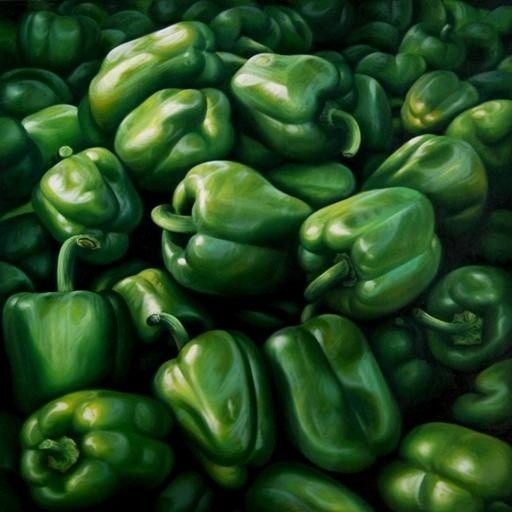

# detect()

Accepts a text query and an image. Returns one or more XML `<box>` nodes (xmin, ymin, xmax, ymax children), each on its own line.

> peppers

<box><xmin>0</xmin><ymin>0</ymin><xmax>510</xmax><ymax>512</ymax></box>
